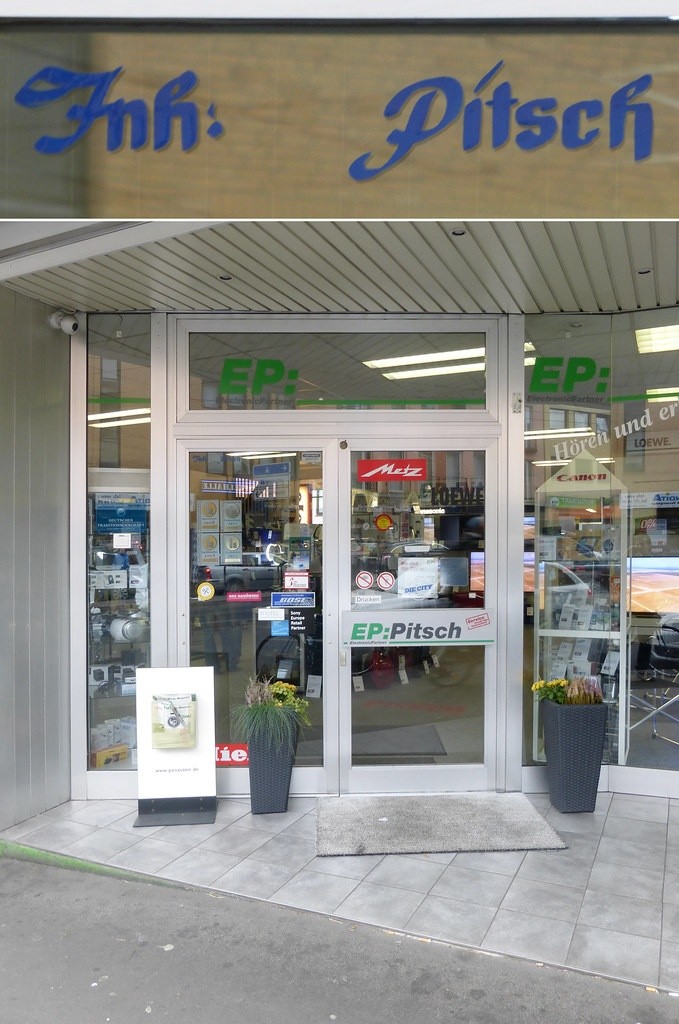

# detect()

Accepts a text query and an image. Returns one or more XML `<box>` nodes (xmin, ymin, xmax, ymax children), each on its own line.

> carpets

<box><xmin>352</xmin><ymin>723</ymin><xmax>447</xmax><ymax>756</ymax></box>
<box><xmin>315</xmin><ymin>793</ymin><xmax>567</xmax><ymax>856</ymax></box>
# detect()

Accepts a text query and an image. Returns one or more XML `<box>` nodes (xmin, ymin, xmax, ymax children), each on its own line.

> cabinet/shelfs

<box><xmin>533</xmin><ymin>449</ymin><xmax>633</xmax><ymax>765</ymax></box>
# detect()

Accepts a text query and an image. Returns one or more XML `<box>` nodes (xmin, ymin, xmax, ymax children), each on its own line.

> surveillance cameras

<box><xmin>61</xmin><ymin>315</ymin><xmax>80</xmax><ymax>335</ymax></box>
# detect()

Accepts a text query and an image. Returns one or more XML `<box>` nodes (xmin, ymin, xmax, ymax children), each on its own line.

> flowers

<box><xmin>531</xmin><ymin>678</ymin><xmax>602</xmax><ymax>706</ymax></box>
<box><xmin>226</xmin><ymin>673</ymin><xmax>312</xmax><ymax>755</ymax></box>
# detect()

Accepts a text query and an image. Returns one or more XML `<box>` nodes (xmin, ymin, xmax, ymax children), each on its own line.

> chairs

<box><xmin>625</xmin><ymin>622</ymin><xmax>679</xmax><ymax>746</ymax></box>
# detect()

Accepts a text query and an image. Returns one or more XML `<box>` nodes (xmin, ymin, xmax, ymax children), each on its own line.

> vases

<box><xmin>543</xmin><ymin>699</ymin><xmax>609</xmax><ymax>813</ymax></box>
<box><xmin>248</xmin><ymin>713</ymin><xmax>301</xmax><ymax>814</ymax></box>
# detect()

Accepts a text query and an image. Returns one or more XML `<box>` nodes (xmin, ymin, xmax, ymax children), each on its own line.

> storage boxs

<box><xmin>89</xmin><ymin>563</ymin><xmax>149</xmax><ymax>590</ymax></box>
<box><xmin>540</xmin><ymin>516</ymin><xmax>620</xmax><ymax>681</ymax></box>
<box><xmin>196</xmin><ymin>500</ymin><xmax>243</xmax><ymax>565</ymax></box>
<box><xmin>351</xmin><ymin>513</ymin><xmax>423</xmax><ymax>544</ymax></box>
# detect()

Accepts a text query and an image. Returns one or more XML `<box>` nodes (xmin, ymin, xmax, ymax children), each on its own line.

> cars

<box><xmin>543</xmin><ymin>564</ymin><xmax>594</xmax><ymax>625</ymax></box>
<box><xmin>93</xmin><ymin>550</ymin><xmax>136</xmax><ymax>600</ymax></box>
<box><xmin>193</xmin><ymin>552</ymin><xmax>293</xmax><ymax>594</ymax></box>
<box><xmin>367</xmin><ymin>539</ymin><xmax>450</xmax><ymax>576</ymax></box>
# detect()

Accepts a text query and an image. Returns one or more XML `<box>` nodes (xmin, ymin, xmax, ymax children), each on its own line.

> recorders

<box><xmin>109</xmin><ymin>617</ymin><xmax>151</xmax><ymax>643</ymax></box>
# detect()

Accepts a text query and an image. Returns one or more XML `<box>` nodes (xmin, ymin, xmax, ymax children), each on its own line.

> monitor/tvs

<box><xmin>524</xmin><ymin>516</ymin><xmax>535</xmax><ymax>549</ymax></box>
<box><xmin>467</xmin><ymin>551</ymin><xmax>535</xmax><ymax>594</ymax></box>
<box><xmin>626</xmin><ymin>556</ymin><xmax>679</xmax><ymax>619</ymax></box>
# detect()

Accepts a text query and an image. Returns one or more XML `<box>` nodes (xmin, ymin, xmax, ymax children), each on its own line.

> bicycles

<box><xmin>602</xmin><ymin>607</ymin><xmax>679</xmax><ymax>678</ymax></box>
<box><xmin>255</xmin><ymin>605</ymin><xmax>385</xmax><ymax>688</ymax></box>
